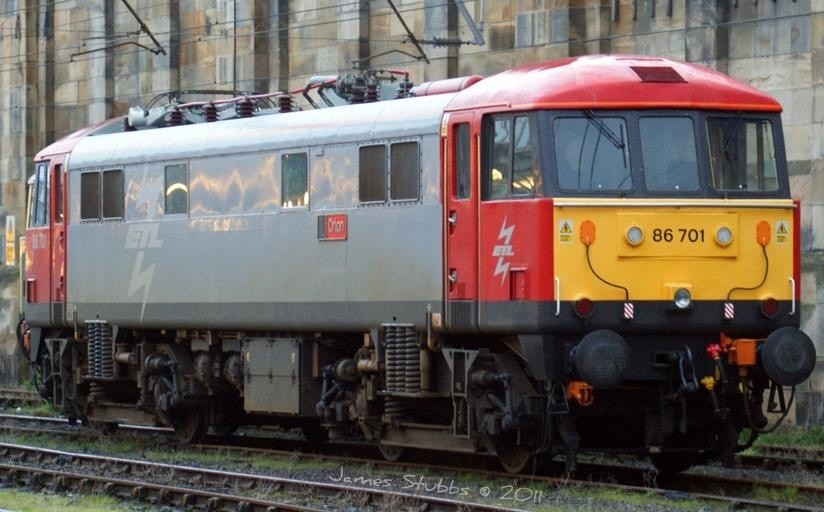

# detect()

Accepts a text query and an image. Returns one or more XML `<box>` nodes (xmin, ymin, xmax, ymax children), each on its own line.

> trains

<box><xmin>18</xmin><ymin>53</ymin><xmax>822</xmax><ymax>477</ymax></box>
